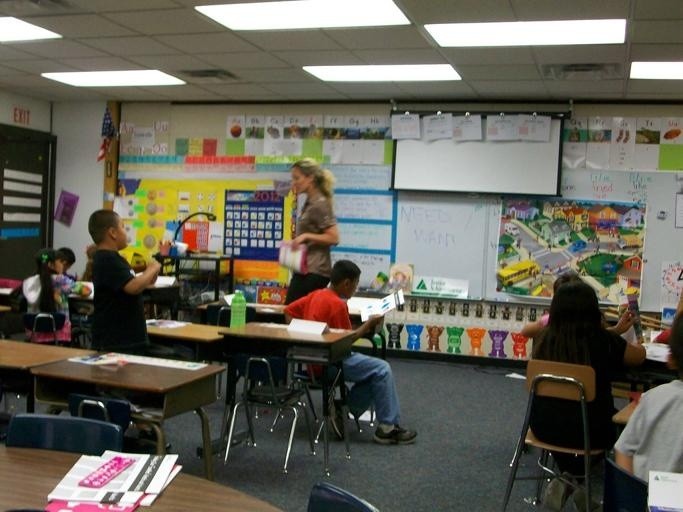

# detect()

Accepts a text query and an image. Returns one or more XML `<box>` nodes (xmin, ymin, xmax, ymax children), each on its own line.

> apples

<box><xmin>231</xmin><ymin>126</ymin><xmax>241</xmax><ymax>138</ymax></box>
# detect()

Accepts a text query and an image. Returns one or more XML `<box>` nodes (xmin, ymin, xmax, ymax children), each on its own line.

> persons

<box><xmin>284</xmin><ymin>156</ymin><xmax>340</xmax><ymax>305</ymax></box>
<box><xmin>88</xmin><ymin>210</ymin><xmax>171</xmax><ymax>450</ymax></box>
<box><xmin>521</xmin><ymin>272</ymin><xmax>646</xmax><ymax>510</ymax></box>
<box><xmin>22</xmin><ymin>247</ymin><xmax>92</xmax><ymax>348</ymax></box>
<box><xmin>613</xmin><ymin>311</ymin><xmax>683</xmax><ymax>489</ymax></box>
<box><xmin>284</xmin><ymin>260</ymin><xmax>418</xmax><ymax>446</ymax></box>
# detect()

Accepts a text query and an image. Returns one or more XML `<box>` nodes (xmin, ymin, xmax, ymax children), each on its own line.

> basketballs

<box><xmin>230</xmin><ymin>124</ymin><xmax>242</xmax><ymax>137</ymax></box>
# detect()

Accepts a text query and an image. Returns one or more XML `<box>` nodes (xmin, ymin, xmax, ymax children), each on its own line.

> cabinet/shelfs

<box><xmin>152</xmin><ymin>251</ymin><xmax>235</xmax><ymax>320</ymax></box>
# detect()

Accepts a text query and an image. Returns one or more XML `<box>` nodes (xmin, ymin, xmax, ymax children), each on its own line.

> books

<box><xmin>279</xmin><ymin>241</ymin><xmax>310</xmax><ymax>276</ymax></box>
<box><xmin>44</xmin><ymin>450</ymin><xmax>184</xmax><ymax>512</ymax></box>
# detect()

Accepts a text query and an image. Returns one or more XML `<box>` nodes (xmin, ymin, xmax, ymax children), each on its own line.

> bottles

<box><xmin>229</xmin><ymin>289</ymin><xmax>246</xmax><ymax>328</ymax></box>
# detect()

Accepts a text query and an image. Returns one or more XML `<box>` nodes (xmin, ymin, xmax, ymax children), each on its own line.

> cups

<box><xmin>170</xmin><ymin>246</ymin><xmax>178</xmax><ymax>256</ymax></box>
<box><xmin>159</xmin><ymin>245</ymin><xmax>170</xmax><ymax>256</ymax></box>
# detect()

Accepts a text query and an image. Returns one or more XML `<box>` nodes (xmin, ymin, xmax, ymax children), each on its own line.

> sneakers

<box><xmin>327</xmin><ymin>398</ymin><xmax>353</xmax><ymax>423</ymax></box>
<box><xmin>543</xmin><ymin>472</ymin><xmax>599</xmax><ymax>510</ymax></box>
<box><xmin>374</xmin><ymin>423</ymin><xmax>418</xmax><ymax>445</ymax></box>
<box><xmin>139</xmin><ymin>429</ymin><xmax>170</xmax><ymax>450</ymax></box>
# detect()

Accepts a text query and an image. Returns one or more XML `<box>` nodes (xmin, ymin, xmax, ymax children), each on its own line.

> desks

<box><xmin>612</xmin><ymin>398</ymin><xmax>637</xmax><ymax>423</ymax></box>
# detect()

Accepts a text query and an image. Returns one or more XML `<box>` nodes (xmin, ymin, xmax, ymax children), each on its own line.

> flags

<box><xmin>96</xmin><ymin>109</ymin><xmax>115</xmax><ymax>162</ymax></box>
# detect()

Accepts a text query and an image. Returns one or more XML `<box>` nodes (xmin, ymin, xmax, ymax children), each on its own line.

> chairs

<box><xmin>603</xmin><ymin>448</ymin><xmax>647</xmax><ymax>510</ymax></box>
<box><xmin>496</xmin><ymin>358</ymin><xmax>612</xmax><ymax>512</ymax></box>
